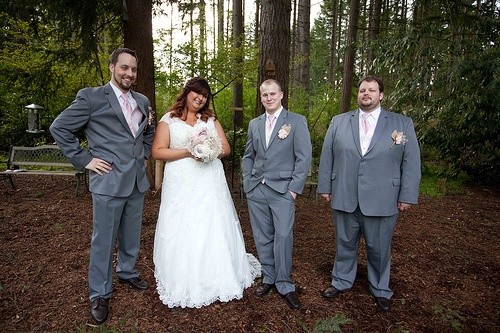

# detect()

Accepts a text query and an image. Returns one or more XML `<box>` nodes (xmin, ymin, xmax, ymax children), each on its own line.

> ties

<box><xmin>121</xmin><ymin>93</ymin><xmax>137</xmax><ymax>138</ymax></box>
<box><xmin>361</xmin><ymin>113</ymin><xmax>371</xmax><ymax>154</ymax></box>
<box><xmin>266</xmin><ymin>115</ymin><xmax>276</xmax><ymax>148</ymax></box>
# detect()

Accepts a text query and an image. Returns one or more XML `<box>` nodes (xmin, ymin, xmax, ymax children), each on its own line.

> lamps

<box><xmin>23</xmin><ymin>101</ymin><xmax>47</xmax><ymax>139</ymax></box>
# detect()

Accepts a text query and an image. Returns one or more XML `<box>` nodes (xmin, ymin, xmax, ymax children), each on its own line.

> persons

<box><xmin>49</xmin><ymin>49</ymin><xmax>156</xmax><ymax>322</ymax></box>
<box><xmin>241</xmin><ymin>79</ymin><xmax>312</xmax><ymax>309</ymax></box>
<box><xmin>152</xmin><ymin>78</ymin><xmax>261</xmax><ymax>310</ymax></box>
<box><xmin>316</xmin><ymin>75</ymin><xmax>421</xmax><ymax>311</ymax></box>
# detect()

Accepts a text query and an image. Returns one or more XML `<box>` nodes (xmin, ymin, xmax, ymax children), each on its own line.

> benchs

<box><xmin>0</xmin><ymin>145</ymin><xmax>90</xmax><ymax>197</ymax></box>
<box><xmin>240</xmin><ymin>164</ymin><xmax>318</xmax><ymax>205</ymax></box>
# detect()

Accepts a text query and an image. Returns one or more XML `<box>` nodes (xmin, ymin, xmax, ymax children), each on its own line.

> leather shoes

<box><xmin>368</xmin><ymin>286</ymin><xmax>390</xmax><ymax>310</ymax></box>
<box><xmin>275</xmin><ymin>285</ymin><xmax>300</xmax><ymax>310</ymax></box>
<box><xmin>255</xmin><ymin>283</ymin><xmax>275</xmax><ymax>297</ymax></box>
<box><xmin>322</xmin><ymin>285</ymin><xmax>350</xmax><ymax>297</ymax></box>
<box><xmin>90</xmin><ymin>298</ymin><xmax>109</xmax><ymax>324</ymax></box>
<box><xmin>118</xmin><ymin>276</ymin><xmax>150</xmax><ymax>290</ymax></box>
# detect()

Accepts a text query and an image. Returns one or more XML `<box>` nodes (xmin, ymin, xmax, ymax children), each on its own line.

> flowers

<box><xmin>148</xmin><ymin>108</ymin><xmax>155</xmax><ymax>125</ymax></box>
<box><xmin>183</xmin><ymin>128</ymin><xmax>222</xmax><ymax>163</ymax></box>
<box><xmin>389</xmin><ymin>129</ymin><xmax>408</xmax><ymax>149</ymax></box>
<box><xmin>276</xmin><ymin>122</ymin><xmax>292</xmax><ymax>139</ymax></box>
